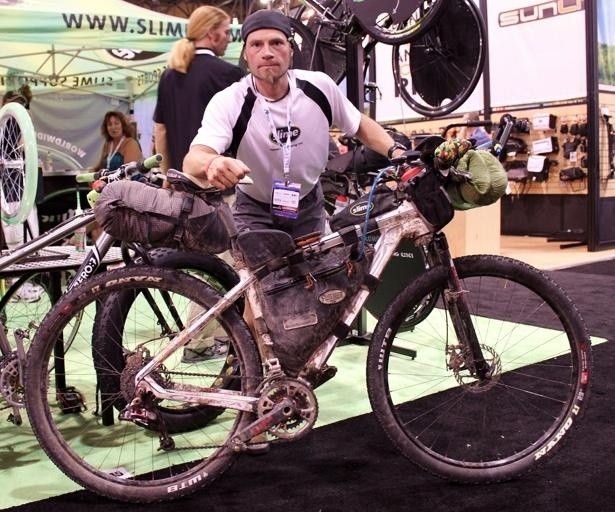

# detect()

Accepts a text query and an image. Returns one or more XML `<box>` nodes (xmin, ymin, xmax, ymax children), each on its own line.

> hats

<box><xmin>241</xmin><ymin>8</ymin><xmax>291</xmax><ymax>42</ymax></box>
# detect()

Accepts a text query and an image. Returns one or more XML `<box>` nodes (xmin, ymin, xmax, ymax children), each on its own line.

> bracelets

<box><xmin>204</xmin><ymin>155</ymin><xmax>225</xmax><ymax>175</ymax></box>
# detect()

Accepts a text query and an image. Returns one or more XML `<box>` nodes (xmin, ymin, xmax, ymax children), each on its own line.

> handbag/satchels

<box><xmin>253</xmin><ymin>236</ymin><xmax>375</xmax><ymax>378</ymax></box>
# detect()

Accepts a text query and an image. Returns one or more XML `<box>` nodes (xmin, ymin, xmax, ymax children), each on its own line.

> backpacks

<box><xmin>94</xmin><ymin>180</ymin><xmax>238</xmax><ymax>254</ymax></box>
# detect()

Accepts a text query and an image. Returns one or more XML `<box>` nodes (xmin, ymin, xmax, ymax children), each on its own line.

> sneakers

<box><xmin>181</xmin><ymin>344</ymin><xmax>229</xmax><ymax>364</ymax></box>
<box><xmin>7</xmin><ymin>285</ymin><xmax>41</xmax><ymax>303</ymax></box>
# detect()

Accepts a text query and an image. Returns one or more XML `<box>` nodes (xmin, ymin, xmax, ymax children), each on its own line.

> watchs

<box><xmin>387</xmin><ymin>141</ymin><xmax>407</xmax><ymax>158</ymax></box>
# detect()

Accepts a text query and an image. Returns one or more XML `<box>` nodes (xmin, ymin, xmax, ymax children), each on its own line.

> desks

<box><xmin>36</xmin><ymin>167</ymin><xmax>96</xmax><ymax>246</ymax></box>
<box><xmin>1</xmin><ymin>243</ymin><xmax>135</xmax><ymax>402</ymax></box>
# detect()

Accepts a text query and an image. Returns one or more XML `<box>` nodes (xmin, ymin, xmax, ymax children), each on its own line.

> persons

<box><xmin>152</xmin><ymin>6</ymin><xmax>245</xmax><ymax>361</ymax></box>
<box><xmin>181</xmin><ymin>8</ymin><xmax>407</xmax><ymax>245</ymax></box>
<box><xmin>446</xmin><ymin>112</ymin><xmax>492</xmax><ymax>148</ymax></box>
<box><xmin>0</xmin><ymin>90</ymin><xmax>27</xmax><ymax>202</ymax></box>
<box><xmin>86</xmin><ymin>110</ymin><xmax>143</xmax><ymax>173</ymax></box>
